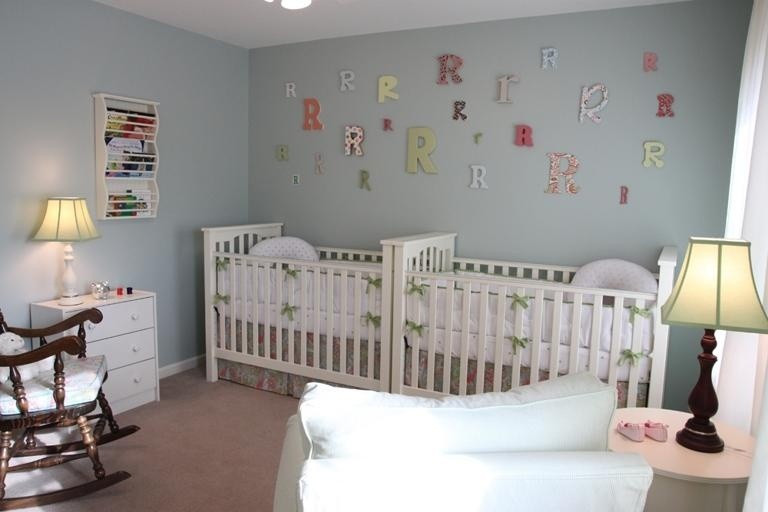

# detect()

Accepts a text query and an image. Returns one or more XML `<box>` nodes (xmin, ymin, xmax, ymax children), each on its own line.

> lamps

<box><xmin>660</xmin><ymin>236</ymin><xmax>768</xmax><ymax>454</ymax></box>
<box><xmin>28</xmin><ymin>197</ymin><xmax>101</xmax><ymax>306</ymax></box>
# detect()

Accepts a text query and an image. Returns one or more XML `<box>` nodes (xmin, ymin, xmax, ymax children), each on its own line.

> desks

<box><xmin>606</xmin><ymin>407</ymin><xmax>756</xmax><ymax>512</ymax></box>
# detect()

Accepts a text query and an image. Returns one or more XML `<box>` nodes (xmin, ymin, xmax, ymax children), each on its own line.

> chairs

<box><xmin>272</xmin><ymin>411</ymin><xmax>653</xmax><ymax>512</ymax></box>
<box><xmin>0</xmin><ymin>307</ymin><xmax>140</xmax><ymax>511</ymax></box>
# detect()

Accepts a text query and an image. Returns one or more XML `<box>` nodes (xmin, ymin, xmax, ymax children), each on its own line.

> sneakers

<box><xmin>616</xmin><ymin>418</ymin><xmax>647</xmax><ymax>442</ymax></box>
<box><xmin>640</xmin><ymin>417</ymin><xmax>671</xmax><ymax>443</ymax></box>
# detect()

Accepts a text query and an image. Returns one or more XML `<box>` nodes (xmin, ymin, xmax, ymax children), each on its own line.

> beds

<box><xmin>201</xmin><ymin>223</ymin><xmax>445</xmax><ymax>400</ymax></box>
<box><xmin>394</xmin><ymin>232</ymin><xmax>678</xmax><ymax>408</ymax></box>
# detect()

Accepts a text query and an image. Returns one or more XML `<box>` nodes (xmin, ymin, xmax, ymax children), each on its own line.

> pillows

<box><xmin>297</xmin><ymin>369</ymin><xmax>621</xmax><ymax>460</ymax></box>
<box><xmin>248</xmin><ymin>236</ymin><xmax>319</xmax><ymax>294</ymax></box>
<box><xmin>566</xmin><ymin>259</ymin><xmax>658</xmax><ymax>309</ymax></box>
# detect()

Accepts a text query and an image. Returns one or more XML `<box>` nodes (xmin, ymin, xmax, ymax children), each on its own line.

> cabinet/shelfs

<box><xmin>30</xmin><ymin>288</ymin><xmax>160</xmax><ymax>425</ymax></box>
